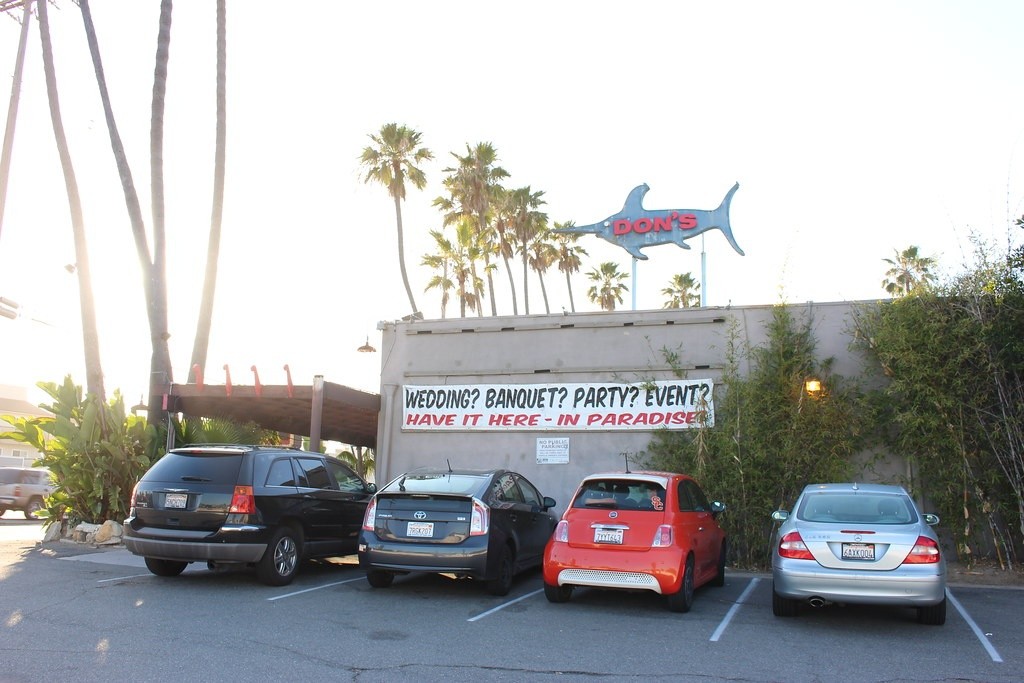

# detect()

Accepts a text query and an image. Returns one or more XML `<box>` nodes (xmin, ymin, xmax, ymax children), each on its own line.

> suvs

<box><xmin>125</xmin><ymin>442</ymin><xmax>378</xmax><ymax>589</ymax></box>
<box><xmin>1</xmin><ymin>468</ymin><xmax>60</xmax><ymax>521</ymax></box>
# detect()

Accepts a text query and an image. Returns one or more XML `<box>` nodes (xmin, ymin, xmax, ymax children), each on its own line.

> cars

<box><xmin>772</xmin><ymin>482</ymin><xmax>948</xmax><ymax>624</ymax></box>
<box><xmin>360</xmin><ymin>459</ymin><xmax>559</xmax><ymax>595</ymax></box>
<box><xmin>544</xmin><ymin>453</ymin><xmax>727</xmax><ymax>616</ymax></box>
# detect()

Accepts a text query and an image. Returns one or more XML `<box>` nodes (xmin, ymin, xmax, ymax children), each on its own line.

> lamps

<box><xmin>804</xmin><ymin>377</ymin><xmax>822</xmax><ymax>397</ymax></box>
